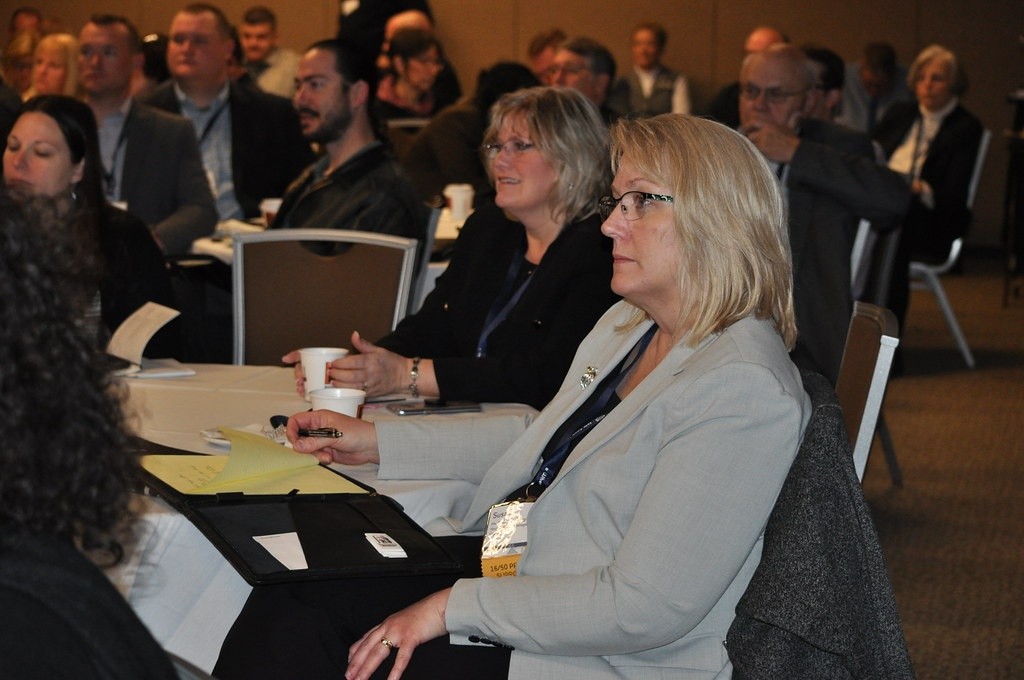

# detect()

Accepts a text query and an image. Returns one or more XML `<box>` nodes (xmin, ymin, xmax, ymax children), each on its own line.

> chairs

<box><xmin>236</xmin><ymin>82</ymin><xmax>992</xmax><ymax>680</ymax></box>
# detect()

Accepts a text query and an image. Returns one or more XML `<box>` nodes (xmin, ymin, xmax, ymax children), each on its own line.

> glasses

<box><xmin>598</xmin><ymin>189</ymin><xmax>675</xmax><ymax>221</ymax></box>
<box><xmin>482</xmin><ymin>136</ymin><xmax>536</xmax><ymax>156</ymax></box>
<box><xmin>737</xmin><ymin>82</ymin><xmax>811</xmax><ymax>105</ymax></box>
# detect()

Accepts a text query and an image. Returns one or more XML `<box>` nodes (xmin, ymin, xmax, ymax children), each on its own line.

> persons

<box><xmin>734</xmin><ymin>51</ymin><xmax>912</xmax><ymax>382</ymax></box>
<box><xmin>868</xmin><ymin>45</ymin><xmax>983</xmax><ymax>378</ymax></box>
<box><xmin>0</xmin><ymin>95</ymin><xmax>175</xmax><ymax>359</ymax></box>
<box><xmin>266</xmin><ymin>39</ymin><xmax>426</xmax><ymax>276</ymax></box>
<box><xmin>0</xmin><ymin>178</ymin><xmax>178</xmax><ymax>680</ymax></box>
<box><xmin>0</xmin><ymin>0</ymin><xmax>919</xmax><ymax>191</ymax></box>
<box><xmin>286</xmin><ymin>113</ymin><xmax>812</xmax><ymax>680</ymax></box>
<box><xmin>132</xmin><ymin>4</ymin><xmax>293</xmax><ymax>222</ymax></box>
<box><xmin>282</xmin><ymin>86</ymin><xmax>625</xmax><ymax>412</ymax></box>
<box><xmin>795</xmin><ymin>48</ymin><xmax>846</xmax><ymax>123</ymax></box>
<box><xmin>70</xmin><ymin>13</ymin><xmax>218</xmax><ymax>257</ymax></box>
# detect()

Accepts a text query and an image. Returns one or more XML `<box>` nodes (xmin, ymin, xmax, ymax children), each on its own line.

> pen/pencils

<box><xmin>299</xmin><ymin>427</ymin><xmax>343</xmax><ymax>438</ymax></box>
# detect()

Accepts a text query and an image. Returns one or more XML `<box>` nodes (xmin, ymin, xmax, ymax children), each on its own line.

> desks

<box><xmin>108</xmin><ymin>357</ymin><xmax>542</xmax><ymax>676</ymax></box>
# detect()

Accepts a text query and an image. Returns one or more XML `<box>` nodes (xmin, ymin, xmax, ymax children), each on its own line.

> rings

<box><xmin>381</xmin><ymin>637</ymin><xmax>392</xmax><ymax>648</ymax></box>
<box><xmin>363</xmin><ymin>382</ymin><xmax>367</xmax><ymax>391</ymax></box>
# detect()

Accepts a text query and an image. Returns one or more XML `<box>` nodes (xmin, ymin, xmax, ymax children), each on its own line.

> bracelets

<box><xmin>409</xmin><ymin>357</ymin><xmax>419</xmax><ymax>398</ymax></box>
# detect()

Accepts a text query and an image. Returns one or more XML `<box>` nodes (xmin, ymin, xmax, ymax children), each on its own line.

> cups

<box><xmin>298</xmin><ymin>347</ymin><xmax>350</xmax><ymax>402</ymax></box>
<box><xmin>443</xmin><ymin>182</ymin><xmax>475</xmax><ymax>223</ymax></box>
<box><xmin>310</xmin><ymin>386</ymin><xmax>366</xmax><ymax>421</ymax></box>
<box><xmin>260</xmin><ymin>197</ymin><xmax>283</xmax><ymax>226</ymax></box>
